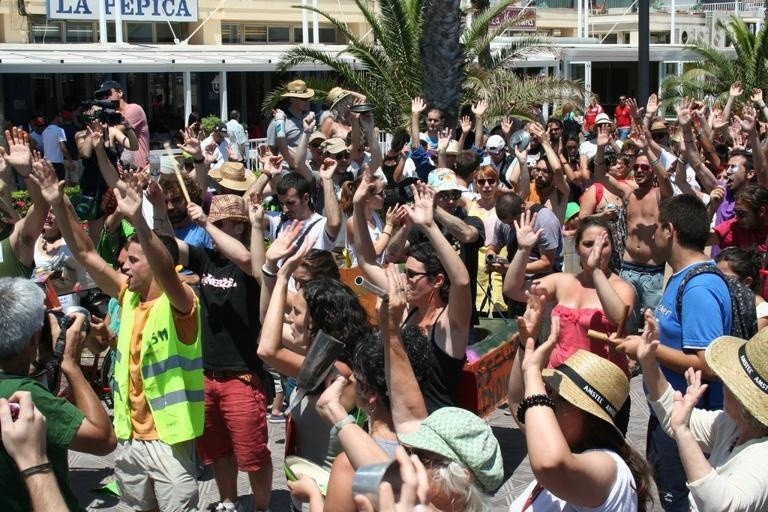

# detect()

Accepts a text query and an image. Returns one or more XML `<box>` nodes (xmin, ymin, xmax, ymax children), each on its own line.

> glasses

<box><xmin>733</xmin><ymin>208</ymin><xmax>752</xmax><ymax>218</ymax></box>
<box><xmin>726</xmin><ymin>164</ymin><xmax>745</xmax><ymax>174</ymax></box>
<box><xmin>632</xmin><ymin>163</ymin><xmax>653</xmax><ymax>172</ymax></box>
<box><xmin>402</xmin><ymin>267</ymin><xmax>436</xmax><ymax>277</ymax></box>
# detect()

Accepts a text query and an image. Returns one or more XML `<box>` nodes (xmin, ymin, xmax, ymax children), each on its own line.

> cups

<box><xmin>296</xmin><ymin>328</ymin><xmax>345</xmax><ymax>393</ymax></box>
<box><xmin>350</xmin><ymin>456</ymin><xmax>404</xmax><ymax>507</ymax></box>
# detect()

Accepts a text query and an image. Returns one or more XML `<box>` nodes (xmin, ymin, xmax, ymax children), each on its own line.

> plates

<box><xmin>348</xmin><ymin>103</ymin><xmax>380</xmax><ymax>115</ymax></box>
<box><xmin>283</xmin><ymin>452</ymin><xmax>332</xmax><ymax>497</ymax></box>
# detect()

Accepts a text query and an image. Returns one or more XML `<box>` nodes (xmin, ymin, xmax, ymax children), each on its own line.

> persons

<box><xmin>0</xmin><ymin>78</ymin><xmax>275</xmax><ymax>512</ymax></box>
<box><xmin>240</xmin><ymin>78</ymin><xmax>767</xmax><ymax>512</ymax></box>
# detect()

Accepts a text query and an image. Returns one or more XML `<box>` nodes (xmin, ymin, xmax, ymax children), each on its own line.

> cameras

<box><xmin>487</xmin><ymin>254</ymin><xmax>508</xmax><ymax>264</ymax></box>
<box><xmin>37</xmin><ymin>312</ymin><xmax>76</xmax><ymax>349</ymax></box>
<box><xmin>568</xmin><ymin>151</ymin><xmax>579</xmax><ymax>163</ymax></box>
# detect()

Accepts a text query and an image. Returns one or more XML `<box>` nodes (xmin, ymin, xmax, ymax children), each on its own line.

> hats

<box><xmin>650</xmin><ymin>122</ymin><xmax>670</xmax><ymax>135</ymax></box>
<box><xmin>541</xmin><ymin>350</ymin><xmax>629</xmax><ymax>440</ymax></box>
<box><xmin>705</xmin><ymin>326</ymin><xmax>768</xmax><ymax>427</ymax></box>
<box><xmin>400</xmin><ymin>408</ymin><xmax>504</xmax><ymax>492</ymax></box>
<box><xmin>206</xmin><ymin>79</ymin><xmax>506</xmax><ymax>225</ymax></box>
<box><xmin>590</xmin><ymin>113</ymin><xmax>613</xmax><ymax>133</ymax></box>
<box><xmin>95</xmin><ymin>80</ymin><xmax>121</xmax><ymax>98</ymax></box>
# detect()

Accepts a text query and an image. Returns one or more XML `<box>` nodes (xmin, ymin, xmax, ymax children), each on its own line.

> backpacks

<box><xmin>677</xmin><ymin>263</ymin><xmax>758</xmax><ymax>341</ymax></box>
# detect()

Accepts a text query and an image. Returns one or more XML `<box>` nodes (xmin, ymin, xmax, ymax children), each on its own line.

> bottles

<box><xmin>274</xmin><ymin>108</ymin><xmax>286</xmax><ymax>139</ymax></box>
